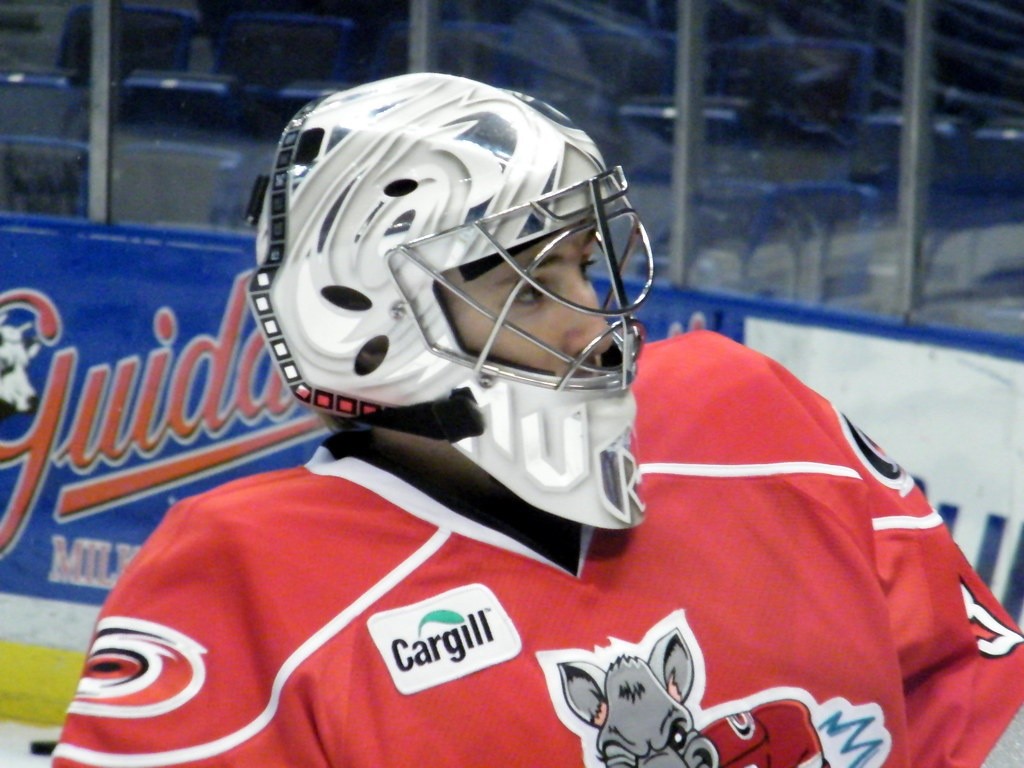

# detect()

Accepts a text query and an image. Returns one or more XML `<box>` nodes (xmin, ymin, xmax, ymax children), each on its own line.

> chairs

<box><xmin>0</xmin><ymin>0</ymin><xmax>1023</xmax><ymax>340</ymax></box>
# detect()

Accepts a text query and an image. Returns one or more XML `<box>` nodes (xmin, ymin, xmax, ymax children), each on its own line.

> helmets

<box><xmin>246</xmin><ymin>71</ymin><xmax>654</xmax><ymax>531</ymax></box>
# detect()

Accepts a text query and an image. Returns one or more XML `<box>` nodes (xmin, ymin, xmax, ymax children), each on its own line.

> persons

<box><xmin>50</xmin><ymin>71</ymin><xmax>1024</xmax><ymax>767</ymax></box>
